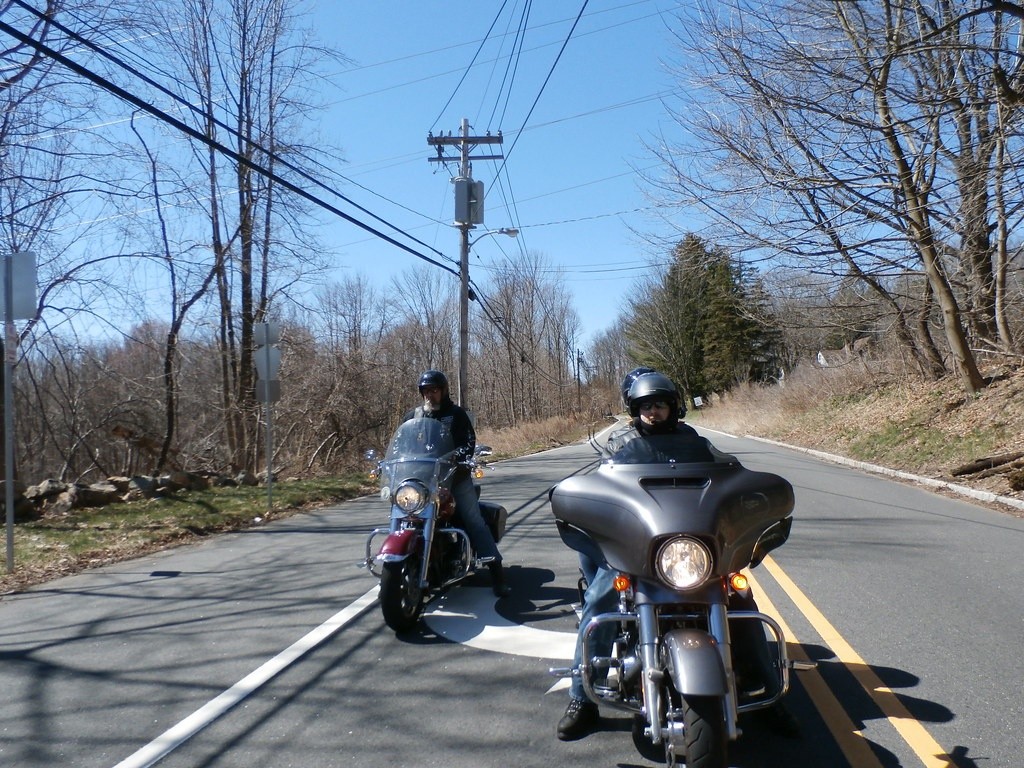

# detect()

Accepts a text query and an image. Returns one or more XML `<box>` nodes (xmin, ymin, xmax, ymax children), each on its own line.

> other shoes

<box><xmin>752</xmin><ymin>702</ymin><xmax>801</xmax><ymax>734</ymax></box>
<box><xmin>493</xmin><ymin>582</ymin><xmax>513</xmax><ymax>597</ymax></box>
<box><xmin>557</xmin><ymin>699</ymin><xmax>599</xmax><ymax>740</ymax></box>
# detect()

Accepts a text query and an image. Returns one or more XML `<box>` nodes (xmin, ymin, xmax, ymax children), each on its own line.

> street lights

<box><xmin>458</xmin><ymin>226</ymin><xmax>519</xmax><ymax>425</ymax></box>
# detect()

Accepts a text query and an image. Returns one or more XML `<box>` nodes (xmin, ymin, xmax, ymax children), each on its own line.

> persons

<box><xmin>557</xmin><ymin>369</ymin><xmax>802</xmax><ymax>739</ymax></box>
<box><xmin>391</xmin><ymin>370</ymin><xmax>512</xmax><ymax>596</ymax></box>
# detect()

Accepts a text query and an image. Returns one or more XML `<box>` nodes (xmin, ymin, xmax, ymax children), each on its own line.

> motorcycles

<box><xmin>356</xmin><ymin>417</ymin><xmax>507</xmax><ymax>632</ymax></box>
<box><xmin>545</xmin><ymin>436</ymin><xmax>795</xmax><ymax>768</ymax></box>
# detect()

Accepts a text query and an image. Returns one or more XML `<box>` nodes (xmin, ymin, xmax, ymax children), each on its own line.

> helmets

<box><xmin>417</xmin><ymin>370</ymin><xmax>449</xmax><ymax>402</ymax></box>
<box><xmin>626</xmin><ymin>372</ymin><xmax>681</xmax><ymax>417</ymax></box>
<box><xmin>622</xmin><ymin>367</ymin><xmax>660</xmax><ymax>409</ymax></box>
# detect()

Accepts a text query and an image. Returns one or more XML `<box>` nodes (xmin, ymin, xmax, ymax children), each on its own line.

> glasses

<box><xmin>421</xmin><ymin>387</ymin><xmax>439</xmax><ymax>395</ymax></box>
<box><xmin>639</xmin><ymin>400</ymin><xmax>669</xmax><ymax>410</ymax></box>
